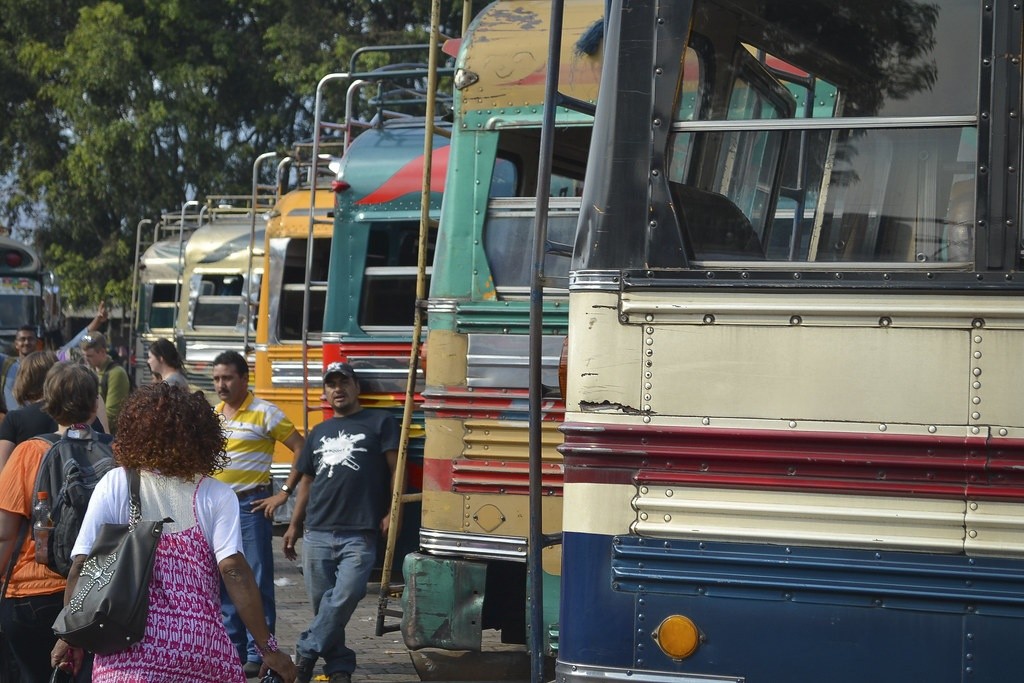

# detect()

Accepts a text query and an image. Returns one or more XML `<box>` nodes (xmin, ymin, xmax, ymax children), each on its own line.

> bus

<box><xmin>528</xmin><ymin>1</ymin><xmax>1024</xmax><ymax>682</ymax></box>
<box><xmin>374</xmin><ymin>1</ymin><xmax>984</xmax><ymax>683</ymax></box>
<box><xmin>0</xmin><ymin>236</ymin><xmax>69</xmax><ymax>374</ymax></box>
<box><xmin>98</xmin><ymin>45</ymin><xmax>575</xmax><ymax>548</ymax></box>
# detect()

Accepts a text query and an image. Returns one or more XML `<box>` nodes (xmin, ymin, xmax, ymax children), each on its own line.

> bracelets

<box><xmin>259</xmin><ymin>632</ymin><xmax>277</xmax><ymax>656</ymax></box>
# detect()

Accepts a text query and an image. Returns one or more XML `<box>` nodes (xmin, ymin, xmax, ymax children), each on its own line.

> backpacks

<box><xmin>29</xmin><ymin>423</ymin><xmax>121</xmax><ymax>579</ymax></box>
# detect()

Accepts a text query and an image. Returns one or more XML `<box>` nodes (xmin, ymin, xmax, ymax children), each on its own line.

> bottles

<box><xmin>33</xmin><ymin>491</ymin><xmax>54</xmax><ymax>564</ymax></box>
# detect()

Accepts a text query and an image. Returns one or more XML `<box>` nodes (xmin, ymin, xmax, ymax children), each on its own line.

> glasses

<box><xmin>82</xmin><ymin>334</ymin><xmax>96</xmax><ymax>345</ymax></box>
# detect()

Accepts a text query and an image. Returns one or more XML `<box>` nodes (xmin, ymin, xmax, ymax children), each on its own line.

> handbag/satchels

<box><xmin>51</xmin><ymin>467</ymin><xmax>173</xmax><ymax>655</ymax></box>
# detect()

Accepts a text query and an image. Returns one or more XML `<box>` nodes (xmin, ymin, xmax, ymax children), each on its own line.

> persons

<box><xmin>0</xmin><ymin>300</ymin><xmax>107</xmax><ymax>429</ymax></box>
<box><xmin>147</xmin><ymin>338</ymin><xmax>190</xmax><ymax>392</ymax></box>
<box><xmin>282</xmin><ymin>361</ymin><xmax>409</xmax><ymax>683</ymax></box>
<box><xmin>0</xmin><ymin>355</ymin><xmax>118</xmax><ymax>683</ymax></box>
<box><xmin>108</xmin><ymin>343</ymin><xmax>134</xmax><ymax>390</ymax></box>
<box><xmin>212</xmin><ymin>350</ymin><xmax>306</xmax><ymax>678</ymax></box>
<box><xmin>50</xmin><ymin>379</ymin><xmax>297</xmax><ymax>683</ymax></box>
<box><xmin>81</xmin><ymin>331</ymin><xmax>128</xmax><ymax>436</ymax></box>
<box><xmin>0</xmin><ymin>349</ymin><xmax>105</xmax><ymax>479</ymax></box>
<box><xmin>66</xmin><ymin>347</ymin><xmax>88</xmax><ymax>366</ymax></box>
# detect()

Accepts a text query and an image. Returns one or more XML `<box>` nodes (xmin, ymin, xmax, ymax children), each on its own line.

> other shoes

<box><xmin>294</xmin><ymin>647</ymin><xmax>316</xmax><ymax>683</ymax></box>
<box><xmin>242</xmin><ymin>661</ymin><xmax>261</xmax><ymax>678</ymax></box>
<box><xmin>329</xmin><ymin>671</ymin><xmax>351</xmax><ymax>683</ymax></box>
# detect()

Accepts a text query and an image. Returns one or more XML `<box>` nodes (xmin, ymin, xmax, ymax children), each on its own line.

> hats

<box><xmin>323</xmin><ymin>363</ymin><xmax>356</xmax><ymax>385</ymax></box>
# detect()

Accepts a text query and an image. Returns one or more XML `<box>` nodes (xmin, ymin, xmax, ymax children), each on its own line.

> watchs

<box><xmin>281</xmin><ymin>484</ymin><xmax>292</xmax><ymax>495</ymax></box>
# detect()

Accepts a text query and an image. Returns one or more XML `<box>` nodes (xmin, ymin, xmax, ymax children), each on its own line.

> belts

<box><xmin>236</xmin><ymin>484</ymin><xmax>267</xmax><ymax>500</ymax></box>
